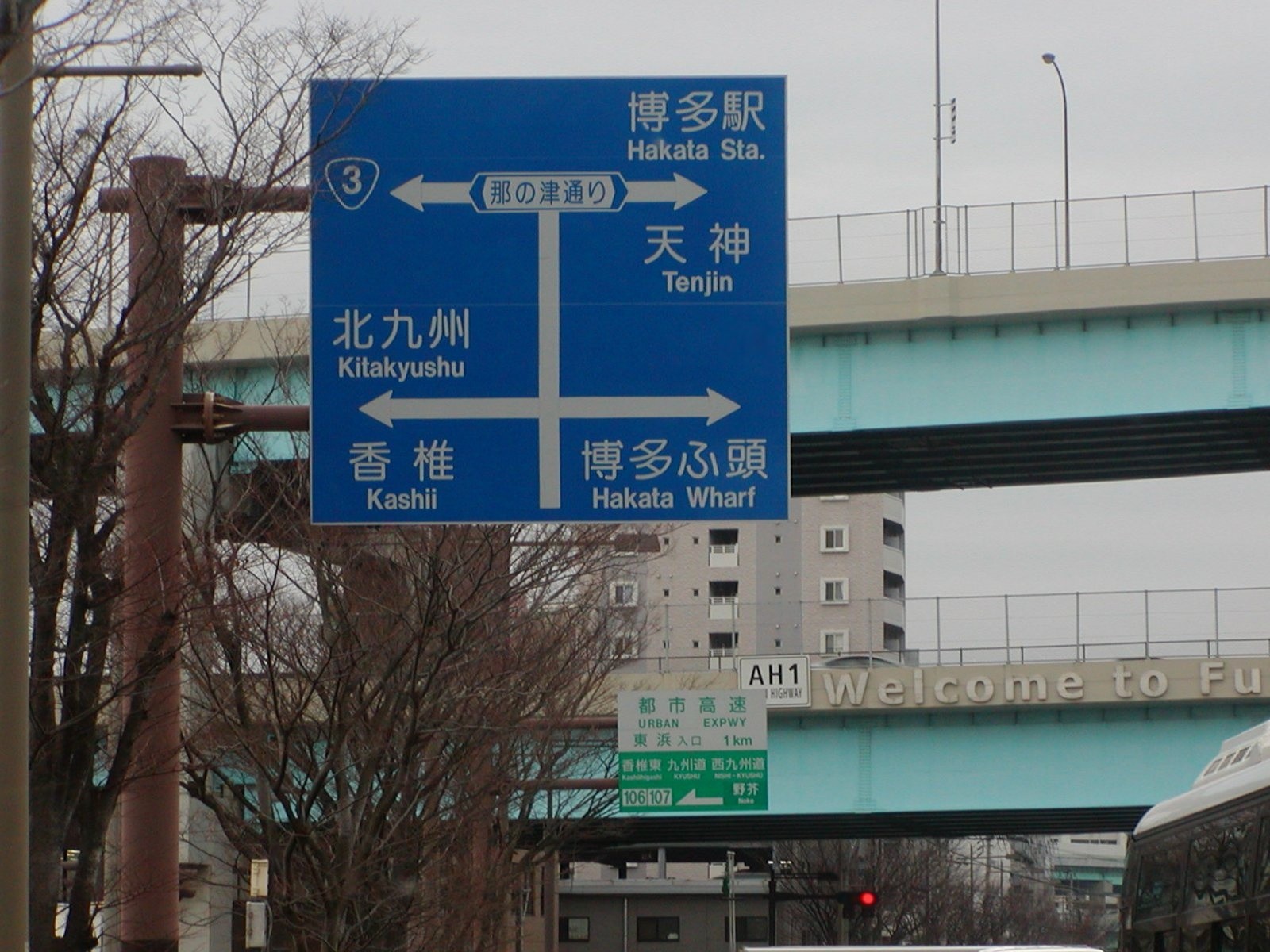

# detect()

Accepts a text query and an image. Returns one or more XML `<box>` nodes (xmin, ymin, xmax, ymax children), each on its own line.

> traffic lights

<box><xmin>860</xmin><ymin>893</ymin><xmax>875</xmax><ymax>917</ymax></box>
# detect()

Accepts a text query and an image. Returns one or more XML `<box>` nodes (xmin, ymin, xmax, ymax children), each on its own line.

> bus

<box><xmin>1121</xmin><ymin>720</ymin><xmax>1270</xmax><ymax>952</ymax></box>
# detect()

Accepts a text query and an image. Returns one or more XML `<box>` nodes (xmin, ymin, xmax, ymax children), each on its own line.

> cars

<box><xmin>810</xmin><ymin>654</ymin><xmax>905</xmax><ymax>668</ymax></box>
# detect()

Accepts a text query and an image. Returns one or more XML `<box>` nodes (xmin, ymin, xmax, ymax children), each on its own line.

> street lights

<box><xmin>1040</xmin><ymin>53</ymin><xmax>1073</xmax><ymax>267</ymax></box>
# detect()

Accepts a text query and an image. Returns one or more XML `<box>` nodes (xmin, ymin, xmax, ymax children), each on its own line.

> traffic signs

<box><xmin>619</xmin><ymin>689</ymin><xmax>770</xmax><ymax>811</ymax></box>
<box><xmin>304</xmin><ymin>75</ymin><xmax>797</xmax><ymax>520</ymax></box>
